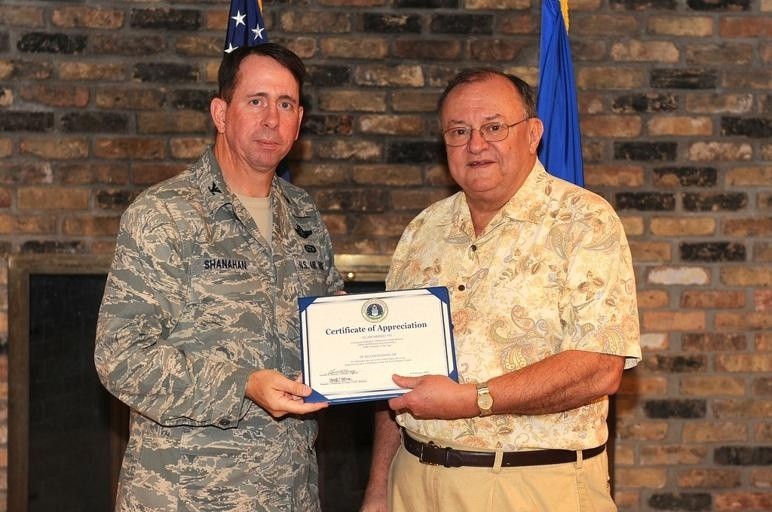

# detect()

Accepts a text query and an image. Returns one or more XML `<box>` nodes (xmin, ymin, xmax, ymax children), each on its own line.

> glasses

<box><xmin>441</xmin><ymin>115</ymin><xmax>532</xmax><ymax>147</ymax></box>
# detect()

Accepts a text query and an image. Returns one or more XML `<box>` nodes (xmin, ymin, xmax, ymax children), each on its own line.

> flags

<box><xmin>227</xmin><ymin>0</ymin><xmax>267</xmax><ymax>48</ymax></box>
<box><xmin>538</xmin><ymin>0</ymin><xmax>583</xmax><ymax>185</ymax></box>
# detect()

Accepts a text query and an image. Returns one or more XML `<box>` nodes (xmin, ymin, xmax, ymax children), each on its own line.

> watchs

<box><xmin>476</xmin><ymin>381</ymin><xmax>495</xmax><ymax>417</ymax></box>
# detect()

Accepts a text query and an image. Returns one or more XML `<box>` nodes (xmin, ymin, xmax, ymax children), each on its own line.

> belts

<box><xmin>397</xmin><ymin>424</ymin><xmax>607</xmax><ymax>469</ymax></box>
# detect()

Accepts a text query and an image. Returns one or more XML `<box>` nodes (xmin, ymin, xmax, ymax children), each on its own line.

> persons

<box><xmin>360</xmin><ymin>66</ymin><xmax>643</xmax><ymax>511</ymax></box>
<box><xmin>93</xmin><ymin>42</ymin><xmax>349</xmax><ymax>512</ymax></box>
